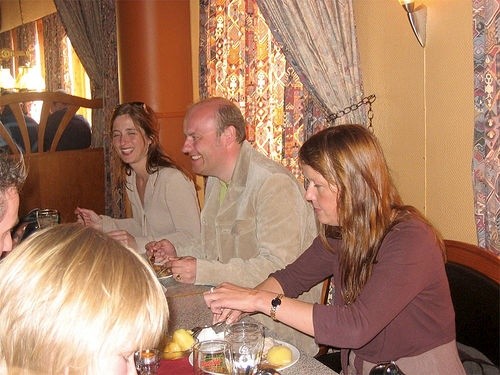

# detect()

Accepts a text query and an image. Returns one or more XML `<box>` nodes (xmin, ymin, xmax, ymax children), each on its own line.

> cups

<box><xmin>37</xmin><ymin>208</ymin><xmax>58</xmax><ymax>228</ymax></box>
<box><xmin>193</xmin><ymin>339</ymin><xmax>233</xmax><ymax>375</ymax></box>
<box><xmin>134</xmin><ymin>349</ymin><xmax>160</xmax><ymax>375</ymax></box>
<box><xmin>224</xmin><ymin>322</ymin><xmax>265</xmax><ymax>375</ymax></box>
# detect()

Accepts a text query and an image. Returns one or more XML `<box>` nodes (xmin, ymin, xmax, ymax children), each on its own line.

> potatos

<box><xmin>159</xmin><ymin>329</ymin><xmax>196</xmax><ymax>359</ymax></box>
<box><xmin>267</xmin><ymin>345</ymin><xmax>292</xmax><ymax>366</ymax></box>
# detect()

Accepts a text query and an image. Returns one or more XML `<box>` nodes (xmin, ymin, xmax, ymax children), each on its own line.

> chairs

<box><xmin>444</xmin><ymin>238</ymin><xmax>500</xmax><ymax>375</ymax></box>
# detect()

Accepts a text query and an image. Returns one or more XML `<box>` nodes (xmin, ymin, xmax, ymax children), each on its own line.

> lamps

<box><xmin>399</xmin><ymin>0</ymin><xmax>427</xmax><ymax>47</ymax></box>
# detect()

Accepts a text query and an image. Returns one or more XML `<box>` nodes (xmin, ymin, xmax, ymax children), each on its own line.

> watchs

<box><xmin>270</xmin><ymin>294</ymin><xmax>283</xmax><ymax>321</ymax></box>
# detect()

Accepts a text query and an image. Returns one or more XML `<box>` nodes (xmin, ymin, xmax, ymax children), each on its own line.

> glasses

<box><xmin>130</xmin><ymin>102</ymin><xmax>147</xmax><ymax>113</ymax></box>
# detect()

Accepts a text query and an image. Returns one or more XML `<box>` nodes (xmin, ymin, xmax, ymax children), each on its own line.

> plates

<box><xmin>189</xmin><ymin>338</ymin><xmax>300</xmax><ymax>375</ymax></box>
<box><xmin>150</xmin><ymin>262</ymin><xmax>173</xmax><ymax>280</ymax></box>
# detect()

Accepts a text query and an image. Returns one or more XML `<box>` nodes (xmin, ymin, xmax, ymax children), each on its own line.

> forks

<box><xmin>190</xmin><ymin>322</ymin><xmax>224</xmax><ymax>337</ymax></box>
<box><xmin>149</xmin><ymin>250</ymin><xmax>156</xmax><ymax>264</ymax></box>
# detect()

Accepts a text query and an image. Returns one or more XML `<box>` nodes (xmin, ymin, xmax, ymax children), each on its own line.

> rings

<box><xmin>210</xmin><ymin>286</ymin><xmax>215</xmax><ymax>293</ymax></box>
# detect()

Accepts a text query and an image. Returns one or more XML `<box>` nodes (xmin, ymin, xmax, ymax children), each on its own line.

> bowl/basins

<box><xmin>159</xmin><ymin>336</ymin><xmax>199</xmax><ymax>360</ymax></box>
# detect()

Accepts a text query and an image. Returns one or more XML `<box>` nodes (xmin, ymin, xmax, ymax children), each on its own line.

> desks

<box><xmin>141</xmin><ymin>252</ymin><xmax>338</xmax><ymax>375</ymax></box>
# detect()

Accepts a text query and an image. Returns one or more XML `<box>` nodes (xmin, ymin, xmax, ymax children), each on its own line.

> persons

<box><xmin>0</xmin><ymin>223</ymin><xmax>170</xmax><ymax>375</ymax></box>
<box><xmin>145</xmin><ymin>97</ymin><xmax>324</xmax><ymax>358</ymax></box>
<box><xmin>43</xmin><ymin>89</ymin><xmax>91</xmax><ymax>151</ymax></box>
<box><xmin>0</xmin><ymin>87</ymin><xmax>39</xmax><ymax>153</ymax></box>
<box><xmin>75</xmin><ymin>101</ymin><xmax>200</xmax><ymax>255</ymax></box>
<box><xmin>203</xmin><ymin>124</ymin><xmax>466</xmax><ymax>375</ymax></box>
<box><xmin>0</xmin><ymin>147</ymin><xmax>27</xmax><ymax>258</ymax></box>
